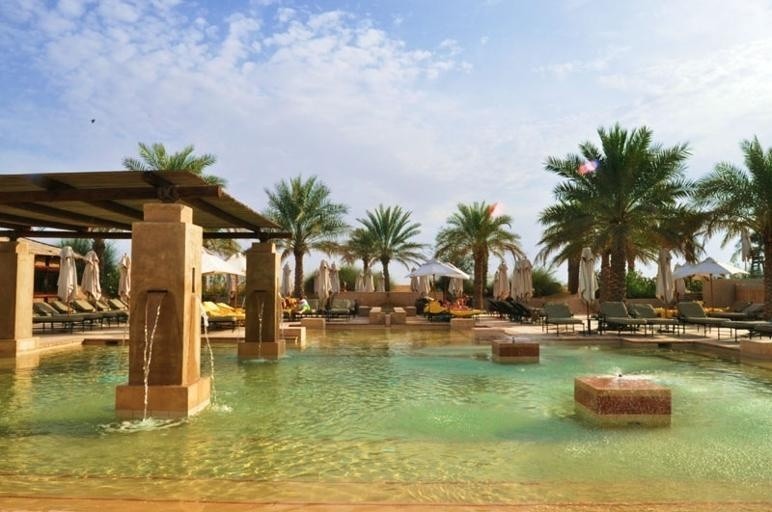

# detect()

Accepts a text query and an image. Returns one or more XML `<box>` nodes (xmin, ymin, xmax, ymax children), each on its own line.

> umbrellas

<box><xmin>81</xmin><ymin>250</ymin><xmax>102</xmax><ymax>311</ymax></box>
<box><xmin>404</xmin><ymin>258</ymin><xmax>470</xmax><ymax>305</ymax></box>
<box><xmin>201</xmin><ymin>247</ymin><xmax>248</xmax><ymax>308</ymax></box>
<box><xmin>656</xmin><ymin>248</ymin><xmax>675</xmax><ymax>317</ymax></box>
<box><xmin>282</xmin><ymin>261</ymin><xmax>293</xmax><ymax>297</ymax></box>
<box><xmin>494</xmin><ymin>257</ymin><xmax>535</xmax><ymax>321</ymax></box>
<box><xmin>313</xmin><ymin>259</ymin><xmax>340</xmax><ymax>315</ymax></box>
<box><xmin>57</xmin><ymin>246</ymin><xmax>78</xmax><ymax>314</ymax></box>
<box><xmin>118</xmin><ymin>253</ymin><xmax>131</xmax><ymax>303</ymax></box>
<box><xmin>354</xmin><ymin>269</ymin><xmax>385</xmax><ymax>293</ymax></box>
<box><xmin>578</xmin><ymin>247</ymin><xmax>599</xmax><ymax>333</ymax></box>
<box><xmin>674</xmin><ymin>262</ymin><xmax>685</xmax><ymax>302</ymax></box>
<box><xmin>673</xmin><ymin>256</ymin><xmax>750</xmax><ymax>311</ymax></box>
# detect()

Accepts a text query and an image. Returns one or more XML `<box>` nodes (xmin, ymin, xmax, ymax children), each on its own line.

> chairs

<box><xmin>544</xmin><ymin>302</ymin><xmax>586</xmax><ymax>337</ymax></box>
<box><xmin>201</xmin><ymin>301</ymin><xmax>246</xmax><ymax>332</ymax></box>
<box><xmin>598</xmin><ymin>301</ymin><xmax>679</xmax><ymax>338</ymax></box>
<box><xmin>720</xmin><ymin>312</ymin><xmax>772</xmax><ymax>343</ymax></box>
<box><xmin>677</xmin><ymin>301</ymin><xmax>731</xmax><ymax>338</ymax></box>
<box><xmin>705</xmin><ymin>302</ymin><xmax>765</xmax><ymax>339</ymax></box>
<box><xmin>281</xmin><ymin>297</ymin><xmax>357</xmax><ymax>323</ymax></box>
<box><xmin>32</xmin><ymin>296</ymin><xmax>130</xmax><ymax>334</ymax></box>
<box><xmin>423</xmin><ymin>299</ymin><xmax>482</xmax><ymax>324</ymax></box>
